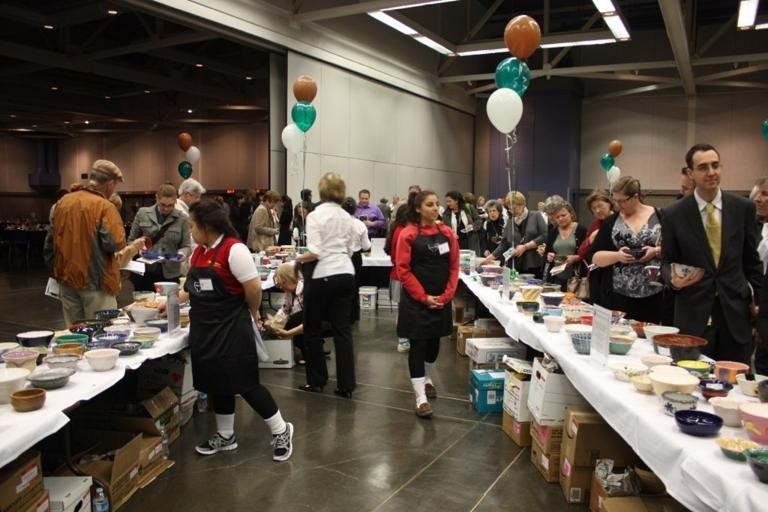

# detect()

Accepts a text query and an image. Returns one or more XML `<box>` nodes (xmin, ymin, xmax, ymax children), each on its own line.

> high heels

<box><xmin>299</xmin><ymin>380</ymin><xmax>325</xmax><ymax>392</ymax></box>
<box><xmin>334</xmin><ymin>386</ymin><xmax>352</xmax><ymax>397</ymax></box>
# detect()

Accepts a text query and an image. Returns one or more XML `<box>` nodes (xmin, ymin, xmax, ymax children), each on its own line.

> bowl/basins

<box><xmin>129</xmin><ymin>277</ymin><xmax>189</xmax><ymax>307</ymax></box>
<box><xmin>1</xmin><ymin>307</ymin><xmax>169</xmax><ymax>409</ymax></box>
<box><xmin>251</xmin><ymin>246</ymin><xmax>305</xmax><ymax>279</ymax></box>
<box><xmin>460</xmin><ymin>249</ymin><xmax>628</xmax><ymax>331</ymax></box>
<box><xmin>625</xmin><ymin>248</ymin><xmax>647</xmax><ymax>257</ymax></box>
<box><xmin>670</xmin><ymin>263</ymin><xmax>705</xmax><ymax>281</ymax></box>
<box><xmin>143</xmin><ymin>251</ymin><xmax>182</xmax><ymax>262</ymax></box>
<box><xmin>569</xmin><ymin>323</ymin><xmax>767</xmax><ymax>483</ymax></box>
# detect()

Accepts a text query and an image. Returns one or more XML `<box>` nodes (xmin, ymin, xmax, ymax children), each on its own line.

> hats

<box><xmin>91</xmin><ymin>159</ymin><xmax>124</xmax><ymax>183</ymax></box>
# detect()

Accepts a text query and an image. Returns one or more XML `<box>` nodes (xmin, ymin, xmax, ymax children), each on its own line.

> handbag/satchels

<box><xmin>566</xmin><ymin>258</ymin><xmax>591</xmax><ymax>298</ymax></box>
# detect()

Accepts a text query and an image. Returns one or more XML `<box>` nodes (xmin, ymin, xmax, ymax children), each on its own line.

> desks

<box><xmin>253</xmin><ymin>252</ymin><xmax>304</xmax><ymax>291</ymax></box>
<box><xmin>1</xmin><ymin>307</ymin><xmax>192</xmax><ymax>467</ymax></box>
<box><xmin>454</xmin><ymin>257</ymin><xmax>766</xmax><ymax>512</ymax></box>
<box><xmin>357</xmin><ymin>256</ymin><xmax>393</xmax><ymax>269</ymax></box>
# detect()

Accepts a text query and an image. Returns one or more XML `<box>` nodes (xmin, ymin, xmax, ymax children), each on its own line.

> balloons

<box><xmin>485</xmin><ymin>15</ymin><xmax>541</xmax><ymax>135</ymax></box>
<box><xmin>177</xmin><ymin>132</ymin><xmax>202</xmax><ymax>180</ymax></box>
<box><xmin>281</xmin><ymin>75</ymin><xmax>318</xmax><ymax>158</ymax></box>
<box><xmin>599</xmin><ymin>140</ymin><xmax>624</xmax><ymax>184</ymax></box>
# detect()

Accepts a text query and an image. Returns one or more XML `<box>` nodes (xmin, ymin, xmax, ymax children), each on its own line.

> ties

<box><xmin>706</xmin><ymin>203</ymin><xmax>721</xmax><ymax>268</ymax></box>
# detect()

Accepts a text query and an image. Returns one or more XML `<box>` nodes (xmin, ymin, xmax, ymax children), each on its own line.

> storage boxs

<box><xmin>262</xmin><ymin>292</ymin><xmax>292</xmax><ymax>308</ymax></box>
<box><xmin>358</xmin><ymin>283</ymin><xmax>376</xmax><ymax>312</ymax></box>
<box><xmin>1</xmin><ymin>413</ymin><xmax>175</xmax><ymax>512</ymax></box>
<box><xmin>452</xmin><ymin>294</ymin><xmax>527</xmax><ymax>415</ymax></box>
<box><xmin>501</xmin><ymin>354</ymin><xmax>590</xmax><ymax>447</ymax></box>
<box><xmin>68</xmin><ymin>347</ymin><xmax>207</xmax><ymax>445</ymax></box>
<box><xmin>258</xmin><ymin>336</ymin><xmax>295</xmax><ymax>368</ymax></box>
<box><xmin>530</xmin><ymin>404</ymin><xmax>690</xmax><ymax>512</ymax></box>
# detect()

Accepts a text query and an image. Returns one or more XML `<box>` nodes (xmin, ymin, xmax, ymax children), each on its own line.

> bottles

<box><xmin>160</xmin><ymin>425</ymin><xmax>170</xmax><ymax>461</ymax></box>
<box><xmin>292</xmin><ymin>226</ymin><xmax>299</xmax><ymax>239</ymax></box>
<box><xmin>167</xmin><ymin>292</ymin><xmax>182</xmax><ymax>333</ymax></box>
<box><xmin>91</xmin><ymin>489</ymin><xmax>110</xmax><ymax>512</ymax></box>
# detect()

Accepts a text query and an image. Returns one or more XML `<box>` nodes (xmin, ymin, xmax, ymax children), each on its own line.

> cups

<box><xmin>180</xmin><ymin>312</ymin><xmax>189</xmax><ymax>328</ymax></box>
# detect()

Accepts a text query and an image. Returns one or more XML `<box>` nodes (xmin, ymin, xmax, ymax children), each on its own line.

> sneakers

<box><xmin>397</xmin><ymin>336</ymin><xmax>410</xmax><ymax>353</ymax></box>
<box><xmin>415</xmin><ymin>400</ymin><xmax>434</xmax><ymax>416</ymax></box>
<box><xmin>195</xmin><ymin>431</ymin><xmax>238</xmax><ymax>455</ymax></box>
<box><xmin>272</xmin><ymin>421</ymin><xmax>294</xmax><ymax>462</ymax></box>
<box><xmin>424</xmin><ymin>378</ymin><xmax>436</xmax><ymax>398</ymax></box>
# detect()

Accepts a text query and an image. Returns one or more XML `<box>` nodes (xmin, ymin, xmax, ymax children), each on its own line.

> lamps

<box><xmin>734</xmin><ymin>0</ymin><xmax>768</xmax><ymax>34</ymax></box>
<box><xmin>370</xmin><ymin>0</ymin><xmax>635</xmax><ymax>58</ymax></box>
